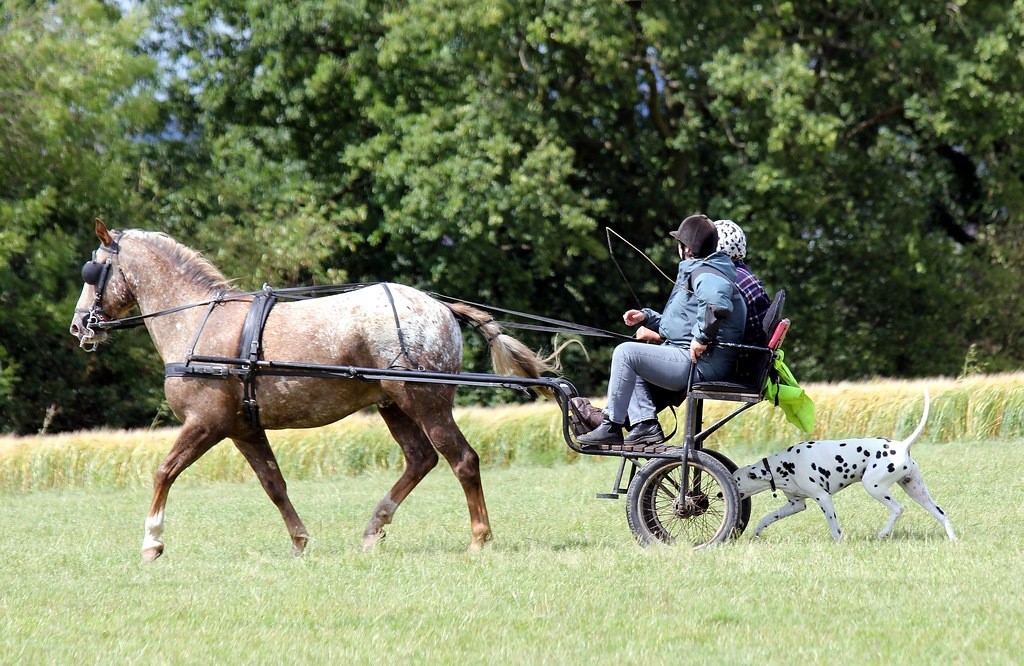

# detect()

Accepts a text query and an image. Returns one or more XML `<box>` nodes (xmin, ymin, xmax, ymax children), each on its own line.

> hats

<box><xmin>713</xmin><ymin>219</ymin><xmax>747</xmax><ymax>260</ymax></box>
<box><xmin>668</xmin><ymin>214</ymin><xmax>719</xmax><ymax>256</ymax></box>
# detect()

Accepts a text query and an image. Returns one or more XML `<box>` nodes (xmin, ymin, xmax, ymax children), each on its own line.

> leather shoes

<box><xmin>623</xmin><ymin>421</ymin><xmax>665</xmax><ymax>446</ymax></box>
<box><xmin>575</xmin><ymin>423</ymin><xmax>625</xmax><ymax>446</ymax></box>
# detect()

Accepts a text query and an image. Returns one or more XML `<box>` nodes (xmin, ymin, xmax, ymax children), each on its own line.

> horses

<box><xmin>69</xmin><ymin>215</ymin><xmax>591</xmax><ymax>564</ymax></box>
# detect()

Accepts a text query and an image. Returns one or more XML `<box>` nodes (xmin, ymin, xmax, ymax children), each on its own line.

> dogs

<box><xmin>716</xmin><ymin>386</ymin><xmax>961</xmax><ymax>543</ymax></box>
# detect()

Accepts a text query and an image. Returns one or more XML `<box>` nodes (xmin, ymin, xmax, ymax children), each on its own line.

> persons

<box><xmin>572</xmin><ymin>219</ymin><xmax>770</xmax><ymax>426</ymax></box>
<box><xmin>576</xmin><ymin>214</ymin><xmax>745</xmax><ymax>447</ymax></box>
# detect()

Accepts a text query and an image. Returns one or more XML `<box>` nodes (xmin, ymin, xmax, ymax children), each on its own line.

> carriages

<box><xmin>69</xmin><ymin>216</ymin><xmax>793</xmax><ymax>565</ymax></box>
